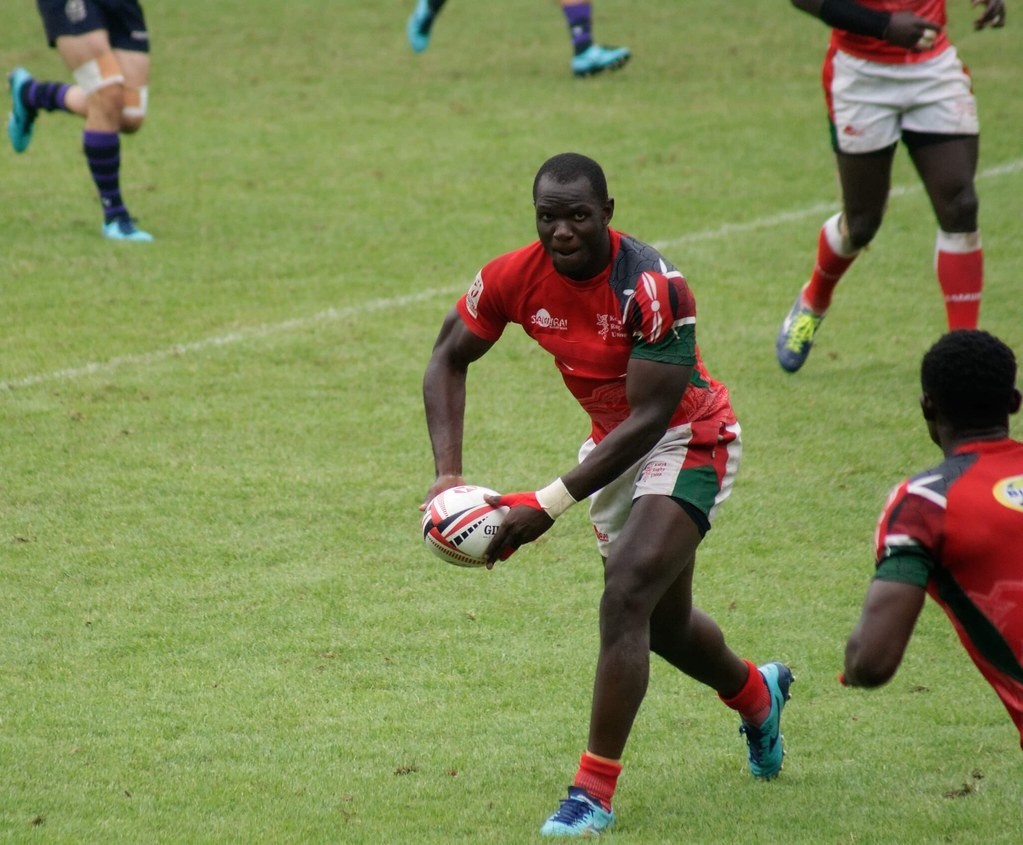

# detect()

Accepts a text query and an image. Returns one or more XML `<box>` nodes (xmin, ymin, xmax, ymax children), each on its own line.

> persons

<box><xmin>419</xmin><ymin>152</ymin><xmax>796</xmax><ymax>835</ymax></box>
<box><xmin>839</xmin><ymin>329</ymin><xmax>1023</xmax><ymax>750</ymax></box>
<box><xmin>394</xmin><ymin>1</ymin><xmax>632</xmax><ymax>74</ymax></box>
<box><xmin>8</xmin><ymin>1</ymin><xmax>150</xmax><ymax>242</ymax></box>
<box><xmin>776</xmin><ymin>0</ymin><xmax>1006</xmax><ymax>372</ymax></box>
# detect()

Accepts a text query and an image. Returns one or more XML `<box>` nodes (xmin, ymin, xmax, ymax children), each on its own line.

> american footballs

<box><xmin>419</xmin><ymin>484</ymin><xmax>512</xmax><ymax>568</ymax></box>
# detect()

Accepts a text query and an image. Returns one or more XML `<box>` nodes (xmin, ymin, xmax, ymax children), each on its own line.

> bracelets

<box><xmin>535</xmin><ymin>477</ymin><xmax>577</xmax><ymax>520</ymax></box>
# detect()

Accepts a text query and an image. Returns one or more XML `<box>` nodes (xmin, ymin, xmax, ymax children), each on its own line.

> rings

<box><xmin>915</xmin><ymin>28</ymin><xmax>937</xmax><ymax>49</ymax></box>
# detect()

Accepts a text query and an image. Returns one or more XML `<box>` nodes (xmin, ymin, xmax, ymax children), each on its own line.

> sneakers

<box><xmin>739</xmin><ymin>663</ymin><xmax>792</xmax><ymax>781</ymax></box>
<box><xmin>6</xmin><ymin>68</ymin><xmax>36</xmax><ymax>153</ymax></box>
<box><xmin>102</xmin><ymin>212</ymin><xmax>152</xmax><ymax>243</ymax></box>
<box><xmin>569</xmin><ymin>42</ymin><xmax>633</xmax><ymax>76</ymax></box>
<box><xmin>776</xmin><ymin>281</ymin><xmax>829</xmax><ymax>373</ymax></box>
<box><xmin>407</xmin><ymin>0</ymin><xmax>441</xmax><ymax>52</ymax></box>
<box><xmin>538</xmin><ymin>786</ymin><xmax>614</xmax><ymax>840</ymax></box>
